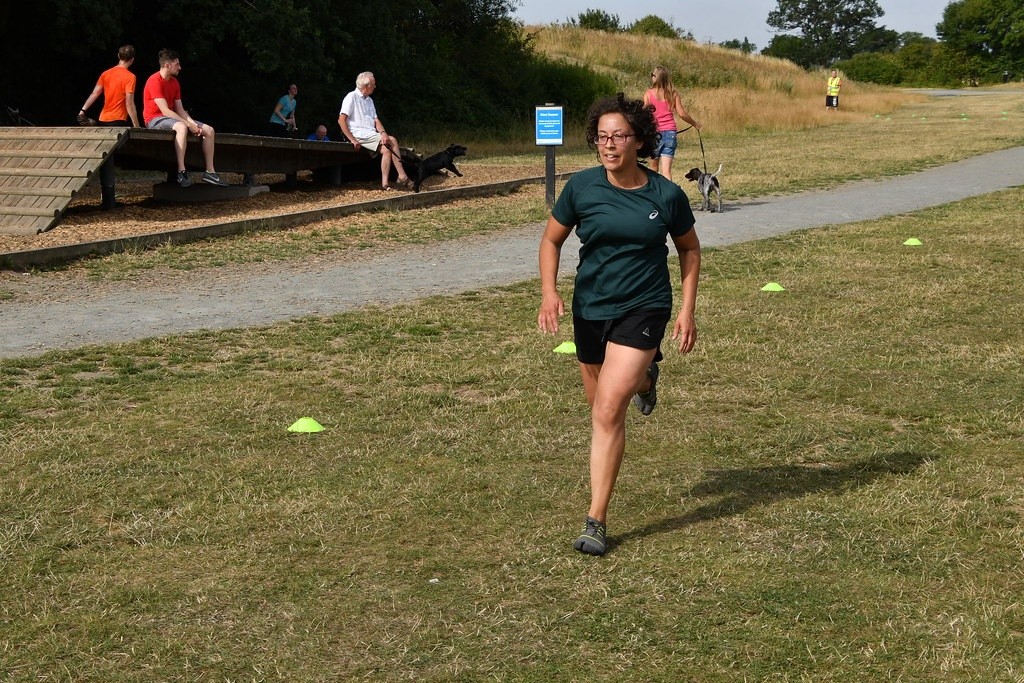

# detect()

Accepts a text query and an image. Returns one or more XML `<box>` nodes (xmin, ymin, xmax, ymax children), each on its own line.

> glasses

<box><xmin>650</xmin><ymin>71</ymin><xmax>656</xmax><ymax>77</ymax></box>
<box><xmin>593</xmin><ymin>132</ymin><xmax>636</xmax><ymax>145</ymax></box>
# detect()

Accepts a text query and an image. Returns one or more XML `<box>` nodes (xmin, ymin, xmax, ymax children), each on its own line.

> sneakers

<box><xmin>175</xmin><ymin>168</ymin><xmax>192</xmax><ymax>187</ymax></box>
<box><xmin>202</xmin><ymin>168</ymin><xmax>229</xmax><ymax>186</ymax></box>
<box><xmin>633</xmin><ymin>360</ymin><xmax>659</xmax><ymax>416</ymax></box>
<box><xmin>572</xmin><ymin>517</ymin><xmax>608</xmax><ymax>555</ymax></box>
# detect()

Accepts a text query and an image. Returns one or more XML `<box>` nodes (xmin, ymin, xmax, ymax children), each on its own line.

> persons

<box><xmin>537</xmin><ymin>92</ymin><xmax>700</xmax><ymax>557</ymax></box>
<box><xmin>77</xmin><ymin>45</ymin><xmax>140</xmax><ymax>128</ymax></box>
<box><xmin>270</xmin><ymin>83</ymin><xmax>298</xmax><ymax>138</ymax></box>
<box><xmin>642</xmin><ymin>66</ymin><xmax>701</xmax><ymax>181</ymax></box>
<box><xmin>825</xmin><ymin>70</ymin><xmax>842</xmax><ymax>112</ymax></box>
<box><xmin>338</xmin><ymin>71</ymin><xmax>415</xmax><ymax>191</ymax></box>
<box><xmin>307</xmin><ymin>125</ymin><xmax>330</xmax><ymax>141</ymax></box>
<box><xmin>142</xmin><ymin>48</ymin><xmax>230</xmax><ymax>187</ymax></box>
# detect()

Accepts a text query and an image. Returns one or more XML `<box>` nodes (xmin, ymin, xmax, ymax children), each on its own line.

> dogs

<box><xmin>398</xmin><ymin>143</ymin><xmax>468</xmax><ymax>193</ymax></box>
<box><xmin>685</xmin><ymin>163</ymin><xmax>723</xmax><ymax>213</ymax></box>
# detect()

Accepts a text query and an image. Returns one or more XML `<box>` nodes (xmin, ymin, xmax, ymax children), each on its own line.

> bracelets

<box><xmin>380</xmin><ymin>131</ymin><xmax>386</xmax><ymax>134</ymax></box>
<box><xmin>81</xmin><ymin>109</ymin><xmax>86</xmax><ymax>114</ymax></box>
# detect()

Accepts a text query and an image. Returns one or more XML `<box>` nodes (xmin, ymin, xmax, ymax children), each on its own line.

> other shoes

<box><xmin>396</xmin><ymin>175</ymin><xmax>420</xmax><ymax>190</ymax></box>
<box><xmin>381</xmin><ymin>184</ymin><xmax>399</xmax><ymax>192</ymax></box>
